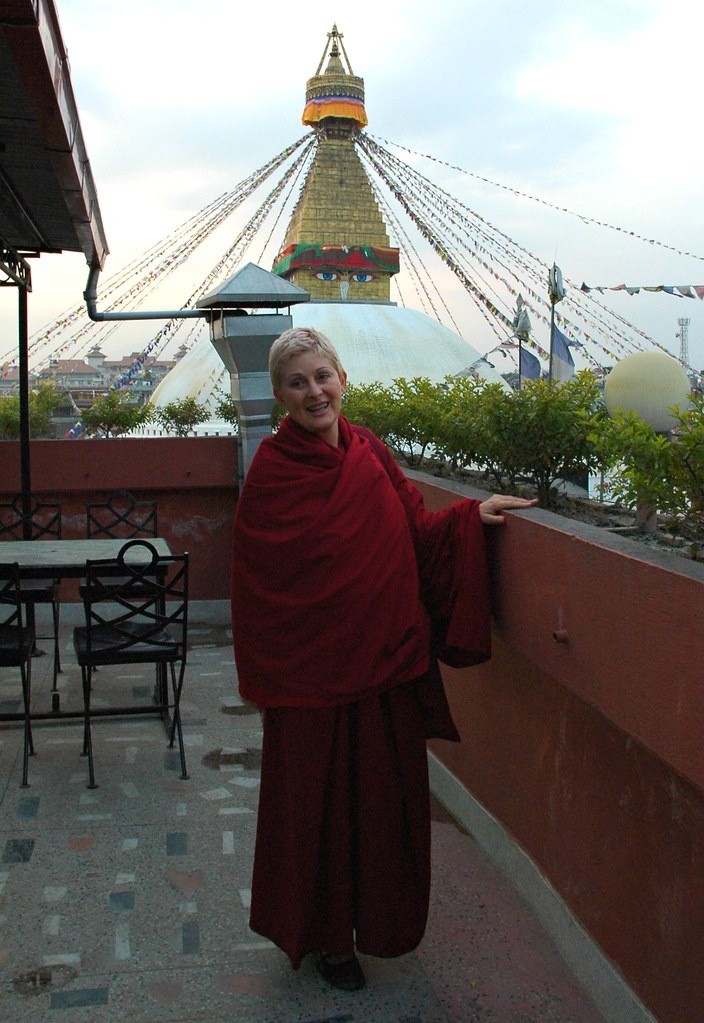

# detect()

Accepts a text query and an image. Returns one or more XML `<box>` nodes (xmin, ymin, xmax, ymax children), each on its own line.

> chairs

<box><xmin>73</xmin><ymin>541</ymin><xmax>190</xmax><ymax>789</ymax></box>
<box><xmin>0</xmin><ymin>561</ymin><xmax>38</xmax><ymax>789</ymax></box>
<box><xmin>83</xmin><ymin>493</ymin><xmax>165</xmax><ymax>671</ymax></box>
<box><xmin>0</xmin><ymin>496</ymin><xmax>63</xmax><ymax>674</ymax></box>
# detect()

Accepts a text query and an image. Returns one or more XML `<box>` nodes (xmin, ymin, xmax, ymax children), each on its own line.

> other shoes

<box><xmin>311</xmin><ymin>948</ymin><xmax>365</xmax><ymax>990</ymax></box>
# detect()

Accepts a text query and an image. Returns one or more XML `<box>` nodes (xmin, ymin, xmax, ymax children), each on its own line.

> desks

<box><xmin>0</xmin><ymin>538</ymin><xmax>175</xmax><ymax>738</ymax></box>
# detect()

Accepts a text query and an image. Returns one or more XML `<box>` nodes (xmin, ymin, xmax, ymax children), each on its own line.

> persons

<box><xmin>227</xmin><ymin>328</ymin><xmax>538</xmax><ymax>992</ymax></box>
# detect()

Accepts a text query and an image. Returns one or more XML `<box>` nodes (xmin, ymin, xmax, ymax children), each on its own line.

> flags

<box><xmin>552</xmin><ymin>322</ymin><xmax>580</xmax><ymax>387</ymax></box>
<box><xmin>0</xmin><ymin>129</ymin><xmax>704</xmax><ymax>442</ymax></box>
<box><xmin>520</xmin><ymin>347</ymin><xmax>540</xmax><ymax>381</ymax></box>
